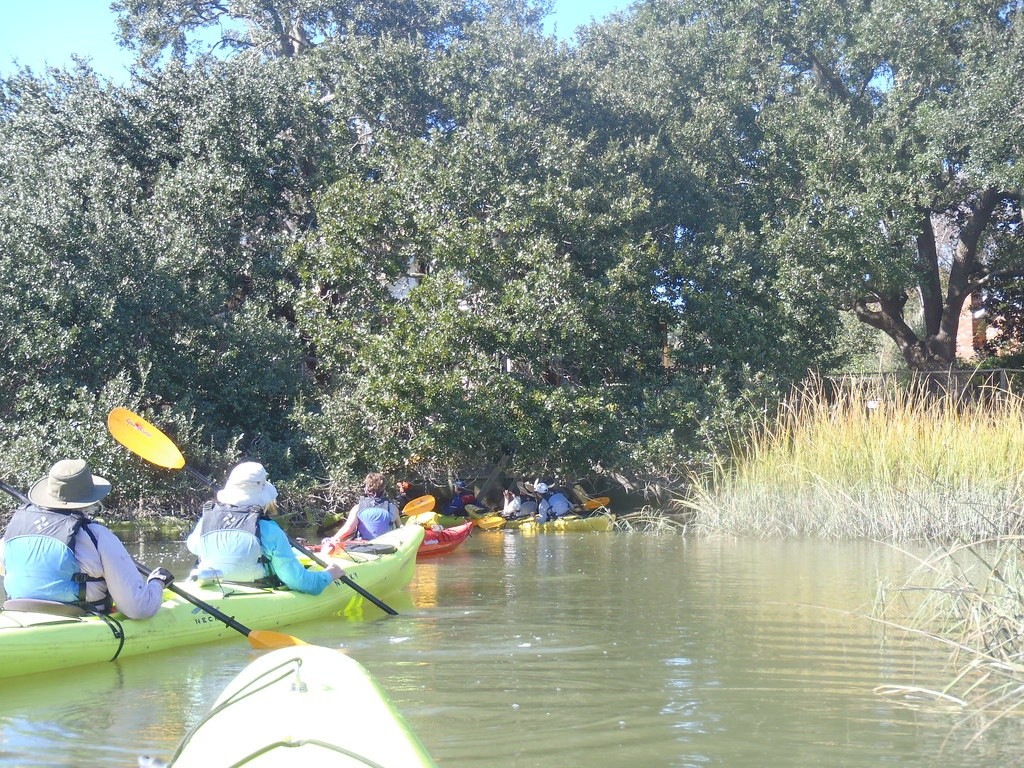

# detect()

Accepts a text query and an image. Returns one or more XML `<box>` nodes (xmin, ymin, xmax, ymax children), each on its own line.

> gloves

<box><xmin>147</xmin><ymin>567</ymin><xmax>174</xmax><ymax>589</ymax></box>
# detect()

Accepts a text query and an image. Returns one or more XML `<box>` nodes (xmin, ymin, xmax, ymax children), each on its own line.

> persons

<box><xmin>499</xmin><ymin>481</ymin><xmax>538</xmax><ymax>518</ymax></box>
<box><xmin>442</xmin><ymin>481</ymin><xmax>489</xmax><ymax>516</ymax></box>
<box><xmin>394</xmin><ymin>480</ymin><xmax>413</xmax><ymax>515</ymax></box>
<box><xmin>184</xmin><ymin>462</ymin><xmax>347</xmax><ymax>595</ymax></box>
<box><xmin>324</xmin><ymin>473</ymin><xmax>402</xmax><ymax>544</ymax></box>
<box><xmin>534</xmin><ymin>482</ymin><xmax>574</xmax><ymax>523</ymax></box>
<box><xmin>0</xmin><ymin>458</ymin><xmax>176</xmax><ymax>620</ymax></box>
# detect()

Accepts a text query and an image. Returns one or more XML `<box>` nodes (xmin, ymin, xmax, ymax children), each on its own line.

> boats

<box><xmin>301</xmin><ymin>507</ymin><xmax>469</xmax><ymax>537</ymax></box>
<box><xmin>88</xmin><ymin>512</ymin><xmax>297</xmax><ymax>542</ymax></box>
<box><xmin>303</xmin><ymin>521</ymin><xmax>474</xmax><ymax>557</ymax></box>
<box><xmin>465</xmin><ymin>503</ymin><xmax>615</xmax><ymax>533</ymax></box>
<box><xmin>1</xmin><ymin>524</ymin><xmax>426</xmax><ymax>678</ymax></box>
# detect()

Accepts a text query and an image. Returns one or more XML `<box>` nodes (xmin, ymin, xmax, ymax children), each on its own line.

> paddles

<box><xmin>108</xmin><ymin>406</ymin><xmax>400</xmax><ymax>616</ymax></box>
<box><xmin>338</xmin><ymin>494</ymin><xmax>435</xmax><ymax>542</ymax></box>
<box><xmin>0</xmin><ymin>481</ymin><xmax>309</xmax><ymax>647</ymax></box>
<box><xmin>477</xmin><ymin>496</ymin><xmax>610</xmax><ymax>530</ymax></box>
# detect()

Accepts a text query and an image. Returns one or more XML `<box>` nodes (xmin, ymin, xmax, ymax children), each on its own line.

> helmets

<box><xmin>453</xmin><ymin>482</ymin><xmax>466</xmax><ymax>490</ymax></box>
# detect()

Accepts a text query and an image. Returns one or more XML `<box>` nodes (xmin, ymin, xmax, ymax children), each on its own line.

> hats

<box><xmin>28</xmin><ymin>459</ymin><xmax>112</xmax><ymax>509</ymax></box>
<box><xmin>217</xmin><ymin>462</ymin><xmax>278</xmax><ymax>507</ymax></box>
<box><xmin>397</xmin><ymin>481</ymin><xmax>413</xmax><ymax>490</ymax></box>
<box><xmin>534</xmin><ymin>482</ymin><xmax>548</xmax><ymax>493</ymax></box>
<box><xmin>517</xmin><ymin>481</ymin><xmax>537</xmax><ymax>498</ymax></box>
